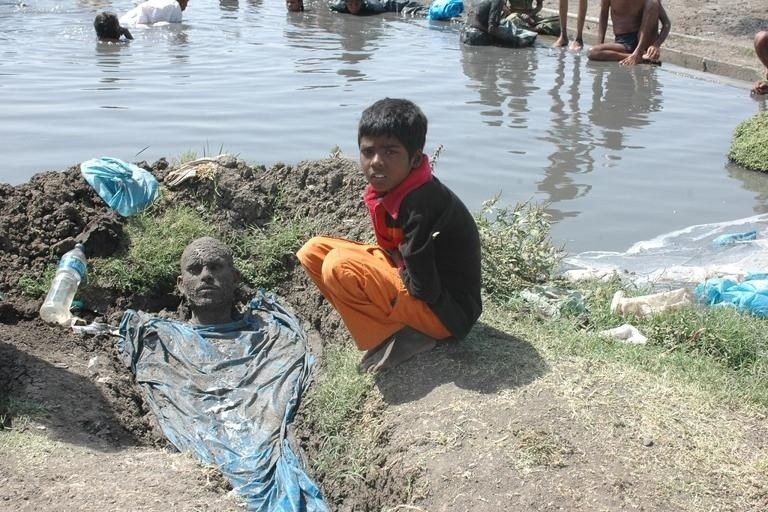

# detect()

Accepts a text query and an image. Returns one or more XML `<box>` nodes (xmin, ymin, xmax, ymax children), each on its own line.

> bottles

<box><xmin>39</xmin><ymin>243</ymin><xmax>86</xmax><ymax>325</ymax></box>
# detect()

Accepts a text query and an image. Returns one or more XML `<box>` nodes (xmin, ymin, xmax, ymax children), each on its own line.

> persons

<box><xmin>747</xmin><ymin>31</ymin><xmax>768</xmax><ymax>97</ymax></box>
<box><xmin>294</xmin><ymin>96</ymin><xmax>483</xmax><ymax>375</ymax></box>
<box><xmin>117</xmin><ymin>235</ymin><xmax>331</xmax><ymax>512</ymax></box>
<box><xmin>552</xmin><ymin>1</ymin><xmax>588</xmax><ymax>51</ymax></box>
<box><xmin>281</xmin><ymin>1</ymin><xmax>306</xmax><ymax>14</ymax></box>
<box><xmin>463</xmin><ymin>1</ymin><xmax>540</xmax><ymax>48</ymax></box>
<box><xmin>116</xmin><ymin>0</ymin><xmax>190</xmax><ymax>28</ymax></box>
<box><xmin>326</xmin><ymin>1</ymin><xmax>409</xmax><ymax>19</ymax></box>
<box><xmin>501</xmin><ymin>1</ymin><xmax>555</xmax><ymax>34</ymax></box>
<box><xmin>92</xmin><ymin>11</ymin><xmax>134</xmax><ymax>43</ymax></box>
<box><xmin>585</xmin><ymin>0</ymin><xmax>671</xmax><ymax>67</ymax></box>
